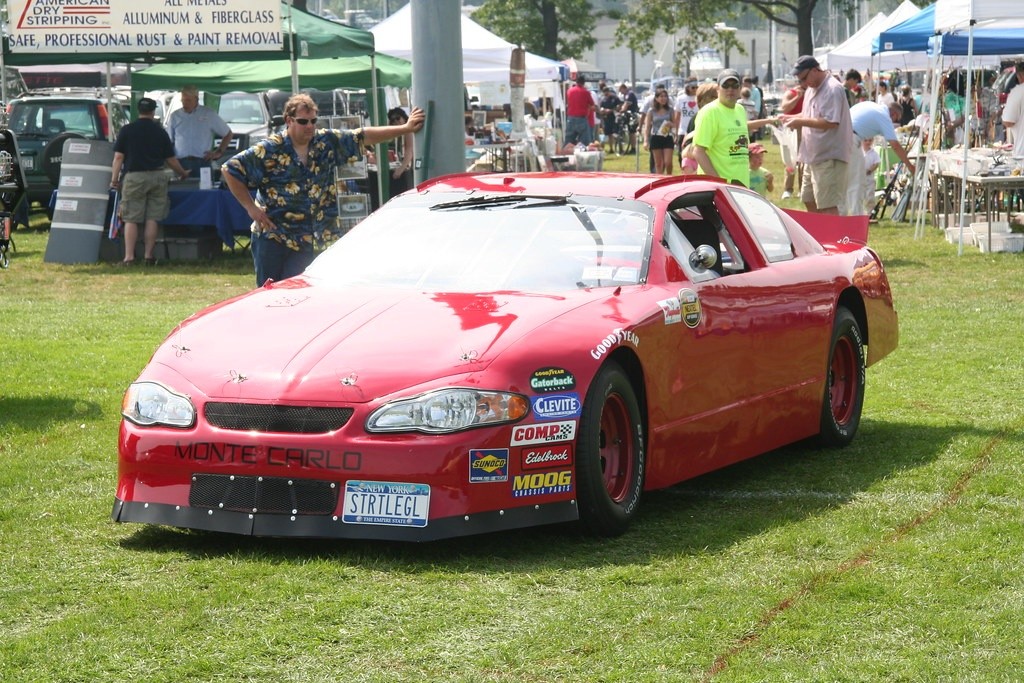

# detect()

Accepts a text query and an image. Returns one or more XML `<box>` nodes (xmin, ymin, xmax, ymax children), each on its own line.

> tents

<box><xmin>1</xmin><ymin>0</ymin><xmax>606</xmax><ymax>207</ymax></box>
<box><xmin>812</xmin><ymin>1</ymin><xmax>1024</xmax><ymax>258</ymax></box>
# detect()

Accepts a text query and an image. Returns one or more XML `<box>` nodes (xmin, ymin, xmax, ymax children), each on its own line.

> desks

<box><xmin>872</xmin><ymin>146</ymin><xmax>928</xmax><ymax>190</ymax></box>
<box><xmin>464</xmin><ymin>109</ymin><xmax>507</xmax><ymax>124</ymax></box>
<box><xmin>461</xmin><ymin>141</ymin><xmax>520</xmax><ymax>174</ymax></box>
<box><xmin>930</xmin><ymin>169</ymin><xmax>1024</xmax><ymax>255</ymax></box>
<box><xmin>50</xmin><ymin>189</ymin><xmax>257</xmax><ymax>253</ymax></box>
<box><xmin>366</xmin><ymin>169</ymin><xmax>413</xmax><ymax>211</ymax></box>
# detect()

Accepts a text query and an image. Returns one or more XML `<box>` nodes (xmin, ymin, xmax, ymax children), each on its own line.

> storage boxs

<box><xmin>930</xmin><ymin>146</ymin><xmax>1024</xmax><ymax>254</ymax></box>
<box><xmin>98</xmin><ymin>224</ymin><xmax>223</xmax><ymax>262</ymax></box>
<box><xmin>476</xmin><ymin>155</ymin><xmax>604</xmax><ymax>170</ymax></box>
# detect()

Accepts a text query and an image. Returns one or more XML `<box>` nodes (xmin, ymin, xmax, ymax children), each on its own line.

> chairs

<box><xmin>674</xmin><ymin>219</ymin><xmax>723</xmax><ymax>274</ymax></box>
<box><xmin>44</xmin><ymin>119</ymin><xmax>64</xmax><ymax>133</ymax></box>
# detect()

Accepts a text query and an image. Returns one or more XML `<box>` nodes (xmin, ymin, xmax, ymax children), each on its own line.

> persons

<box><xmin>567</xmin><ymin>55</ymin><xmax>1024</xmax><ymax>216</ymax></box>
<box><xmin>165</xmin><ymin>85</ymin><xmax>233</xmax><ymax>183</ymax></box>
<box><xmin>221</xmin><ymin>92</ymin><xmax>426</xmax><ymax>288</ymax></box>
<box><xmin>385</xmin><ymin>105</ymin><xmax>415</xmax><ymax>194</ymax></box>
<box><xmin>109</xmin><ymin>98</ymin><xmax>189</xmax><ymax>268</ymax></box>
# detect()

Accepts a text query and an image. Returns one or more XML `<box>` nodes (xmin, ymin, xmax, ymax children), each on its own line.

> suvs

<box><xmin>3</xmin><ymin>89</ymin><xmax>132</xmax><ymax>210</ymax></box>
<box><xmin>160</xmin><ymin>89</ymin><xmax>287</xmax><ymax>150</ymax></box>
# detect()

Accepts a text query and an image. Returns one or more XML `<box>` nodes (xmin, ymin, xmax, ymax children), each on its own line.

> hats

<box><xmin>790</xmin><ymin>55</ymin><xmax>818</xmax><ymax>75</ymax></box>
<box><xmin>748</xmin><ymin>142</ymin><xmax>767</xmax><ymax>154</ymax></box>
<box><xmin>717</xmin><ymin>69</ymin><xmax>740</xmax><ymax>87</ymax></box>
<box><xmin>138</xmin><ymin>98</ymin><xmax>156</xmax><ymax>111</ymax></box>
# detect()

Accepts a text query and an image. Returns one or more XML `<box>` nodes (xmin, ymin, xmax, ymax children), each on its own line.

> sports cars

<box><xmin>108</xmin><ymin>171</ymin><xmax>900</xmax><ymax>538</ymax></box>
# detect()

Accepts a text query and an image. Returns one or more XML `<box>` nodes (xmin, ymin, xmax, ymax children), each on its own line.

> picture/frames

<box><xmin>471</xmin><ymin>111</ymin><xmax>488</xmax><ymax>129</ymax></box>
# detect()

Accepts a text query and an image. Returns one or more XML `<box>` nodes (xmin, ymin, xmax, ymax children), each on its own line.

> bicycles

<box><xmin>612</xmin><ymin>112</ymin><xmax>636</xmax><ymax>157</ymax></box>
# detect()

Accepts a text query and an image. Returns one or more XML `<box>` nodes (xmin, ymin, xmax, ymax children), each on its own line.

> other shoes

<box><xmin>782</xmin><ymin>190</ymin><xmax>790</xmax><ymax>198</ymax></box>
<box><xmin>122</xmin><ymin>260</ymin><xmax>135</xmax><ymax>264</ymax></box>
<box><xmin>628</xmin><ymin>151</ymin><xmax>635</xmax><ymax>154</ymax></box>
<box><xmin>143</xmin><ymin>258</ymin><xmax>152</xmax><ymax>263</ymax></box>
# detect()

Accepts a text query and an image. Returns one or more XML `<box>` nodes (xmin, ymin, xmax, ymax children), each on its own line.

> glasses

<box><xmin>721</xmin><ymin>82</ymin><xmax>739</xmax><ymax>89</ymax></box>
<box><xmin>292</xmin><ymin>115</ymin><xmax>318</xmax><ymax>125</ymax></box>
<box><xmin>390</xmin><ymin>115</ymin><xmax>402</xmax><ymax>122</ymax></box>
<box><xmin>801</xmin><ymin>67</ymin><xmax>812</xmax><ymax>81</ymax></box>
